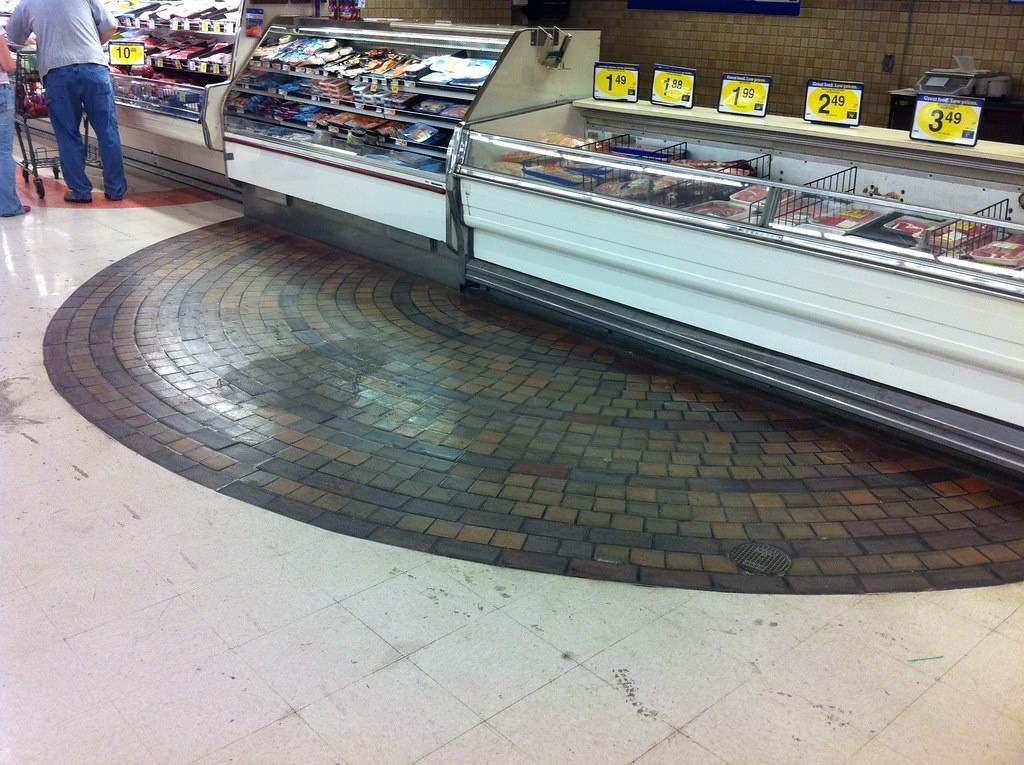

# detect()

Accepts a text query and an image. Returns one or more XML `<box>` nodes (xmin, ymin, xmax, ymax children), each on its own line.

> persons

<box><xmin>5</xmin><ymin>1</ymin><xmax>127</xmax><ymax>203</ymax></box>
<box><xmin>0</xmin><ymin>24</ymin><xmax>31</xmax><ymax>218</ymax></box>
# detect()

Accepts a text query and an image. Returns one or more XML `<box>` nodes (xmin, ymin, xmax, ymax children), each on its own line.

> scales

<box><xmin>914</xmin><ymin>67</ymin><xmax>992</xmax><ymax>97</ymax></box>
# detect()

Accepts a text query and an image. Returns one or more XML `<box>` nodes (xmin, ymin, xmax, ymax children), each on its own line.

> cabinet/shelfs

<box><xmin>221</xmin><ymin>15</ymin><xmax>601</xmax><ymax>293</ymax></box>
<box><xmin>1</xmin><ymin>0</ymin><xmax>319</xmax><ymax>203</ymax></box>
<box><xmin>455</xmin><ymin>93</ymin><xmax>1024</xmax><ymax>491</ymax></box>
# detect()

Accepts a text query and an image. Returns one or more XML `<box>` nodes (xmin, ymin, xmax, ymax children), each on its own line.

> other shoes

<box><xmin>64</xmin><ymin>191</ymin><xmax>92</xmax><ymax>203</ymax></box>
<box><xmin>104</xmin><ymin>191</ymin><xmax>123</xmax><ymax>201</ymax></box>
<box><xmin>23</xmin><ymin>205</ymin><xmax>31</xmax><ymax>212</ymax></box>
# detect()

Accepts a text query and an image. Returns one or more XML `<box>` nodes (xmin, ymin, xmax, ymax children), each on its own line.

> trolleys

<box><xmin>12</xmin><ymin>49</ymin><xmax>106</xmax><ymax>199</ymax></box>
